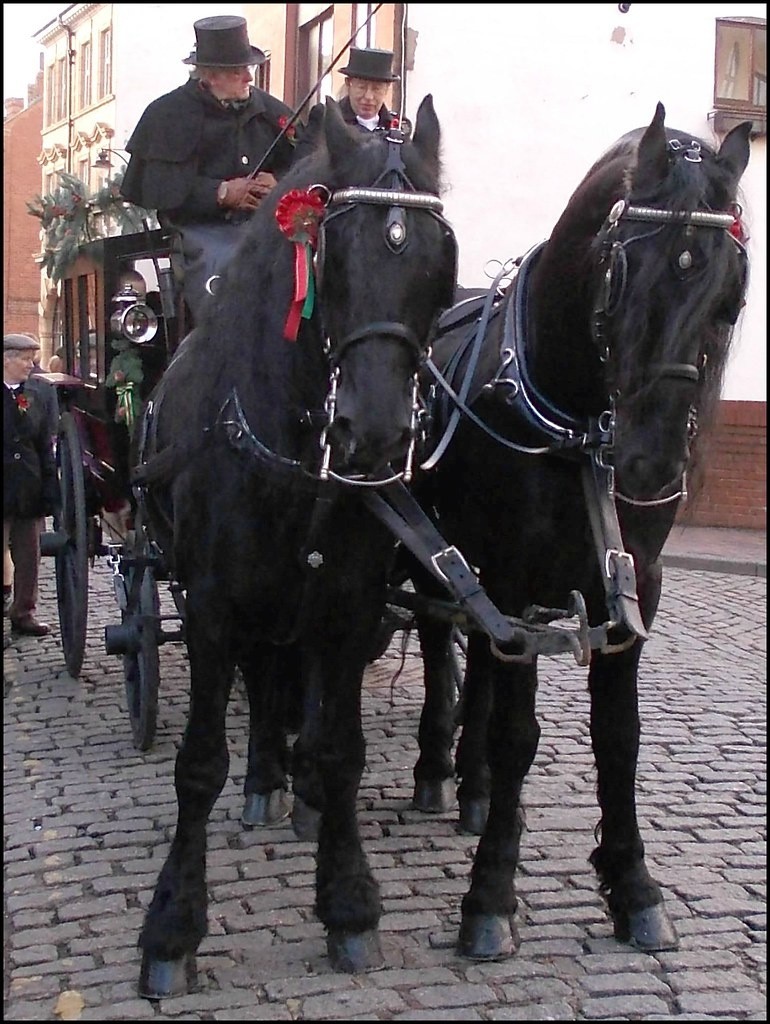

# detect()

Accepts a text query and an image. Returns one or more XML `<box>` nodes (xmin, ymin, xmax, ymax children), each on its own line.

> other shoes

<box><xmin>10</xmin><ymin>614</ymin><xmax>51</xmax><ymax>636</ymax></box>
<box><xmin>3</xmin><ymin>592</ymin><xmax>13</xmax><ymax>616</ymax></box>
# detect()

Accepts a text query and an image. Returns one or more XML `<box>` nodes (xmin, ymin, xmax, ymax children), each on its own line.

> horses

<box><xmin>94</xmin><ymin>95</ymin><xmax>758</xmax><ymax>1004</ymax></box>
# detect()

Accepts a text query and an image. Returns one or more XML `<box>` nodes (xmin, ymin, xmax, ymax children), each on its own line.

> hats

<box><xmin>337</xmin><ymin>47</ymin><xmax>400</xmax><ymax>82</ymax></box>
<box><xmin>3</xmin><ymin>334</ymin><xmax>40</xmax><ymax>350</ymax></box>
<box><xmin>182</xmin><ymin>16</ymin><xmax>266</xmax><ymax>66</ymax></box>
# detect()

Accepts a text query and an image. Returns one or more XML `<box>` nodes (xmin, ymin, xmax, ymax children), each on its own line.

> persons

<box><xmin>116</xmin><ymin>15</ymin><xmax>307</xmax><ymax>325</ymax></box>
<box><xmin>308</xmin><ymin>44</ymin><xmax>412</xmax><ymax>140</ymax></box>
<box><xmin>2</xmin><ymin>267</ymin><xmax>147</xmax><ymax>654</ymax></box>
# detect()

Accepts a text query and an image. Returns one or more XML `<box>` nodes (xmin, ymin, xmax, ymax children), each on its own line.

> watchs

<box><xmin>218</xmin><ymin>182</ymin><xmax>228</xmax><ymax>205</ymax></box>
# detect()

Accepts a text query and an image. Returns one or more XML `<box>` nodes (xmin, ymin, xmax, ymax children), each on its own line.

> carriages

<box><xmin>19</xmin><ymin>93</ymin><xmax>754</xmax><ymax>981</ymax></box>
